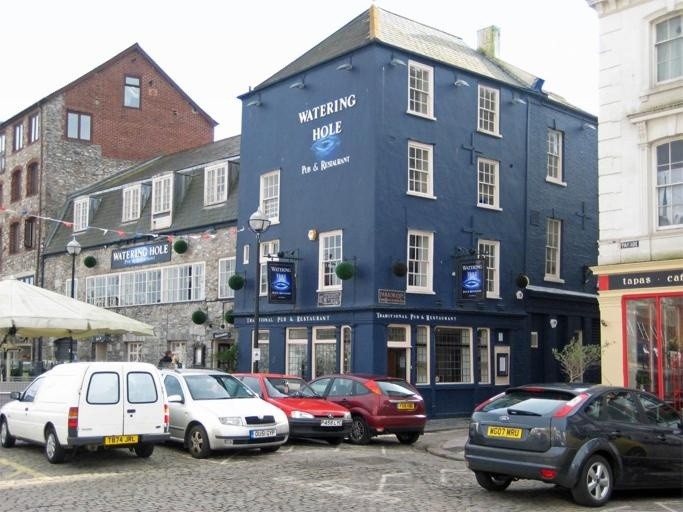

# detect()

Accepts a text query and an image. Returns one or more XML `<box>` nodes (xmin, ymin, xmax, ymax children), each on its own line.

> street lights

<box><xmin>63</xmin><ymin>234</ymin><xmax>81</xmax><ymax>363</ymax></box>
<box><xmin>248</xmin><ymin>205</ymin><xmax>271</xmax><ymax>376</ymax></box>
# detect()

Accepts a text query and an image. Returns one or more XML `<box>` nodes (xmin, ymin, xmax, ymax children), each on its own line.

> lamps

<box><xmin>454</xmin><ymin>79</ymin><xmax>470</xmax><ymax>87</ymax></box>
<box><xmin>512</xmin><ymin>98</ymin><xmax>526</xmax><ymax>105</ymax></box>
<box><xmin>289</xmin><ymin>83</ymin><xmax>305</xmax><ymax>89</ymax></box>
<box><xmin>247</xmin><ymin>100</ymin><xmax>261</xmax><ymax>107</ymax></box>
<box><xmin>391</xmin><ymin>59</ymin><xmax>407</xmax><ymax>66</ymax></box>
<box><xmin>336</xmin><ymin>64</ymin><xmax>352</xmax><ymax>71</ymax></box>
<box><xmin>583</xmin><ymin>122</ymin><xmax>596</xmax><ymax>131</ymax></box>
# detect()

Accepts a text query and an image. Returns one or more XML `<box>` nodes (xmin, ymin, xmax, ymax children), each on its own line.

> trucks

<box><xmin>1</xmin><ymin>361</ymin><xmax>173</xmax><ymax>469</ymax></box>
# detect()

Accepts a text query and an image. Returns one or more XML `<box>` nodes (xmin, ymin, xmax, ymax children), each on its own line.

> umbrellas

<box><xmin>0</xmin><ymin>276</ymin><xmax>157</xmax><ymax>341</ymax></box>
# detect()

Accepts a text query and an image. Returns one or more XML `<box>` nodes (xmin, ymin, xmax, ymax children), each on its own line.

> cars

<box><xmin>531</xmin><ymin>422</ymin><xmax>650</xmax><ymax>467</ymax></box>
<box><xmin>217</xmin><ymin>371</ymin><xmax>354</xmax><ymax>448</ymax></box>
<box><xmin>144</xmin><ymin>367</ymin><xmax>292</xmax><ymax>463</ymax></box>
<box><xmin>287</xmin><ymin>373</ymin><xmax>428</xmax><ymax>448</ymax></box>
<box><xmin>457</xmin><ymin>379</ymin><xmax>683</xmax><ymax>507</ymax></box>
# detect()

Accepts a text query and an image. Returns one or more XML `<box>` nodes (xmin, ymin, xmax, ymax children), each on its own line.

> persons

<box><xmin>156</xmin><ymin>349</ymin><xmax>173</xmax><ymax>371</ymax></box>
